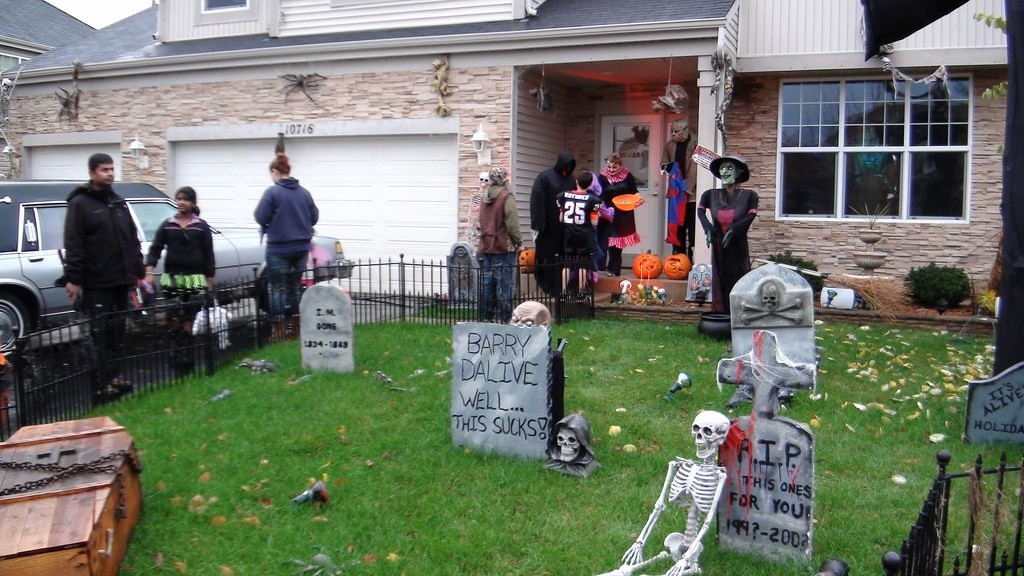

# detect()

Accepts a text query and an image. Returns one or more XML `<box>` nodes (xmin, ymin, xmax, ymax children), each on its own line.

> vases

<box><xmin>696</xmin><ymin>313</ymin><xmax>732</xmax><ymax>341</ymax></box>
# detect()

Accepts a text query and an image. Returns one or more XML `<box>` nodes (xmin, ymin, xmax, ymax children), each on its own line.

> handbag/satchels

<box><xmin>193</xmin><ymin>296</ymin><xmax>232</xmax><ymax>349</ymax></box>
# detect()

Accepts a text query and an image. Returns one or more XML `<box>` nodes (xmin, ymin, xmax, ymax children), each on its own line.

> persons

<box><xmin>254</xmin><ymin>153</ymin><xmax>320</xmax><ymax>341</ymax></box>
<box><xmin>697</xmin><ymin>155</ymin><xmax>758</xmax><ymax>312</ymax></box>
<box><xmin>531</xmin><ymin>151</ymin><xmax>644</xmax><ymax>302</ymax></box>
<box><xmin>596</xmin><ymin>411</ymin><xmax>731</xmax><ymax>576</ymax></box>
<box><xmin>65</xmin><ymin>153</ymin><xmax>146</xmax><ymax>401</ymax></box>
<box><xmin>144</xmin><ymin>186</ymin><xmax>215</xmax><ymax>369</ymax></box>
<box><xmin>660</xmin><ymin>119</ymin><xmax>698</xmax><ymax>262</ymax></box>
<box><xmin>479</xmin><ymin>165</ymin><xmax>525</xmax><ymax>323</ymax></box>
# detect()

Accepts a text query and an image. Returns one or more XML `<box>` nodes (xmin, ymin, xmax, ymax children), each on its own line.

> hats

<box><xmin>490</xmin><ymin>167</ymin><xmax>509</xmax><ymax>186</ymax></box>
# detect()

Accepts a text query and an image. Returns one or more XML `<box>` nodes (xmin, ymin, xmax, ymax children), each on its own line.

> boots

<box><xmin>268</xmin><ymin>319</ymin><xmax>286</xmax><ymax>342</ymax></box>
<box><xmin>285</xmin><ymin>315</ymin><xmax>301</xmax><ymax>340</ymax></box>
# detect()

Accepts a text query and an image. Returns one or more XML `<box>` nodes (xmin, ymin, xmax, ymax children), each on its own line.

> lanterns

<box><xmin>664</xmin><ymin>250</ymin><xmax>691</xmax><ymax>279</ymax></box>
<box><xmin>634</xmin><ymin>250</ymin><xmax>662</xmax><ymax>279</ymax></box>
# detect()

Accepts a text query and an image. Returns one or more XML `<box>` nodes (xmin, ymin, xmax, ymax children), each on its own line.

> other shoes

<box><xmin>109</xmin><ymin>378</ymin><xmax>133</xmax><ymax>392</ymax></box>
<box><xmin>182</xmin><ymin>321</ymin><xmax>193</xmax><ymax>331</ymax></box>
<box><xmin>168</xmin><ymin>321</ymin><xmax>179</xmax><ymax>332</ymax></box>
<box><xmin>93</xmin><ymin>386</ymin><xmax>119</xmax><ymax>400</ymax></box>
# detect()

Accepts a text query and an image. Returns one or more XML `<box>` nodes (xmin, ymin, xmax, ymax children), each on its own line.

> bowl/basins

<box><xmin>612</xmin><ymin>194</ymin><xmax>641</xmax><ymax>211</ymax></box>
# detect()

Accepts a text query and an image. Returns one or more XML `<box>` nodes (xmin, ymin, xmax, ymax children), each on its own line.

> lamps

<box><xmin>129</xmin><ymin>136</ymin><xmax>149</xmax><ymax>170</ymax></box>
<box><xmin>657</xmin><ymin>287</ymin><xmax>666</xmax><ymax>305</ymax></box>
<box><xmin>644</xmin><ymin>285</ymin><xmax>654</xmax><ymax>300</ymax></box>
<box><xmin>469</xmin><ymin>122</ymin><xmax>491</xmax><ymax>165</ymax></box>
<box><xmin>619</xmin><ymin>279</ymin><xmax>632</xmax><ymax>294</ymax></box>
<box><xmin>825</xmin><ymin>289</ymin><xmax>838</xmax><ymax>307</ymax></box>
<box><xmin>2</xmin><ymin>139</ymin><xmax>20</xmax><ymax>171</ymax></box>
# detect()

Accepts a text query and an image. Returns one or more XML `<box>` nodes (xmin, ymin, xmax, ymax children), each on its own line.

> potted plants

<box><xmin>847</xmin><ymin>201</ymin><xmax>891</xmax><ymax>245</ymax></box>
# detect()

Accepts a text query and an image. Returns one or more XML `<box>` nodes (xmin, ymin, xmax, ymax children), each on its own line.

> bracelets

<box><xmin>145</xmin><ymin>272</ymin><xmax>153</xmax><ymax>274</ymax></box>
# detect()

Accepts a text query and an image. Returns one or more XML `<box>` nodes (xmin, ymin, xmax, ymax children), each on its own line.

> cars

<box><xmin>0</xmin><ymin>179</ymin><xmax>355</xmax><ymax>375</ymax></box>
